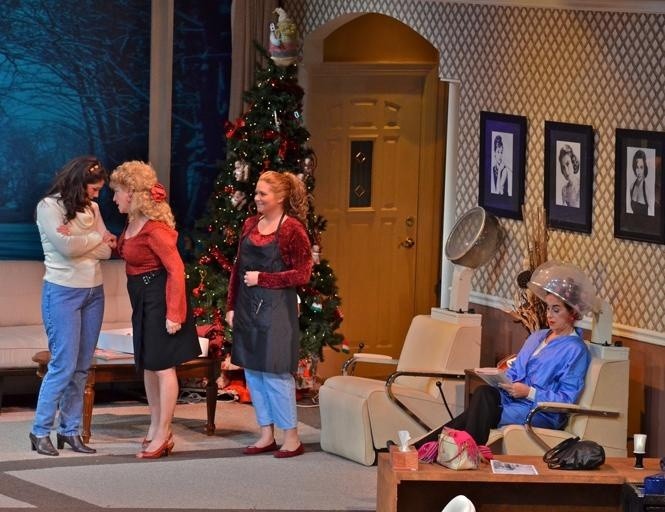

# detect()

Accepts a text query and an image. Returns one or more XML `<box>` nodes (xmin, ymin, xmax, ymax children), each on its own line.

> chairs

<box><xmin>318</xmin><ymin>308</ymin><xmax>484</xmax><ymax>465</ymax></box>
<box><xmin>408</xmin><ymin>351</ymin><xmax>633</xmax><ymax>458</ymax></box>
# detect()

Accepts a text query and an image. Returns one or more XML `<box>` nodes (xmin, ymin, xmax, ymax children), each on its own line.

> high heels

<box><xmin>142</xmin><ymin>436</ymin><xmax>151</xmax><ymax>449</ymax></box>
<box><xmin>57</xmin><ymin>431</ymin><xmax>98</xmax><ymax>453</ymax></box>
<box><xmin>29</xmin><ymin>432</ymin><xmax>59</xmax><ymax>455</ymax></box>
<box><xmin>137</xmin><ymin>433</ymin><xmax>175</xmax><ymax>459</ymax></box>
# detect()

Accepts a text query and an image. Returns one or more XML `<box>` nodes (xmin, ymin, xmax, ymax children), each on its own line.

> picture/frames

<box><xmin>542</xmin><ymin>120</ymin><xmax>594</xmax><ymax>235</ymax></box>
<box><xmin>613</xmin><ymin>127</ymin><xmax>665</xmax><ymax>245</ymax></box>
<box><xmin>477</xmin><ymin>110</ymin><xmax>528</xmax><ymax>221</ymax></box>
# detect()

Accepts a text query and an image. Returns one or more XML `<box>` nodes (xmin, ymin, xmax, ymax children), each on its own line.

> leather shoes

<box><xmin>276</xmin><ymin>441</ymin><xmax>305</xmax><ymax>458</ymax></box>
<box><xmin>242</xmin><ymin>438</ymin><xmax>278</xmax><ymax>455</ymax></box>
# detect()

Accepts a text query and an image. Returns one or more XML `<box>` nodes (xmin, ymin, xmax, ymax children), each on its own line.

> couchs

<box><xmin>0</xmin><ymin>259</ymin><xmax>134</xmax><ymax>373</ymax></box>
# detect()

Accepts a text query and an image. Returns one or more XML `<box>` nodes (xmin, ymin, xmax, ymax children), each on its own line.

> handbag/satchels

<box><xmin>543</xmin><ymin>437</ymin><xmax>606</xmax><ymax>470</ymax></box>
<box><xmin>436</xmin><ymin>425</ymin><xmax>479</xmax><ymax>471</ymax></box>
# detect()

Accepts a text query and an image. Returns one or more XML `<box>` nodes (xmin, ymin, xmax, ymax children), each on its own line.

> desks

<box><xmin>375</xmin><ymin>446</ymin><xmax>625</xmax><ymax>511</ymax></box>
<box><xmin>464</xmin><ymin>365</ymin><xmax>490</xmax><ymax>410</ymax></box>
<box><xmin>605</xmin><ymin>456</ymin><xmax>665</xmax><ymax>484</ymax></box>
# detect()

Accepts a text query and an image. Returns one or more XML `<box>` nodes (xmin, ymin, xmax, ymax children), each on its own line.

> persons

<box><xmin>492</xmin><ymin>135</ymin><xmax>510</xmax><ymax>195</ymax></box>
<box><xmin>29</xmin><ymin>156</ymin><xmax>111</xmax><ymax>456</ymax></box>
<box><xmin>558</xmin><ymin>144</ymin><xmax>580</xmax><ymax>208</ymax></box>
<box><xmin>225</xmin><ymin>171</ymin><xmax>313</xmax><ymax>459</ymax></box>
<box><xmin>102</xmin><ymin>161</ymin><xmax>203</xmax><ymax>460</ymax></box>
<box><xmin>630</xmin><ymin>150</ymin><xmax>651</xmax><ymax>215</ymax></box>
<box><xmin>386</xmin><ymin>278</ymin><xmax>589</xmax><ymax>461</ymax></box>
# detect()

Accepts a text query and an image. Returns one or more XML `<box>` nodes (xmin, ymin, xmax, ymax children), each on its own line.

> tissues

<box><xmin>388</xmin><ymin>430</ymin><xmax>419</xmax><ymax>471</ymax></box>
